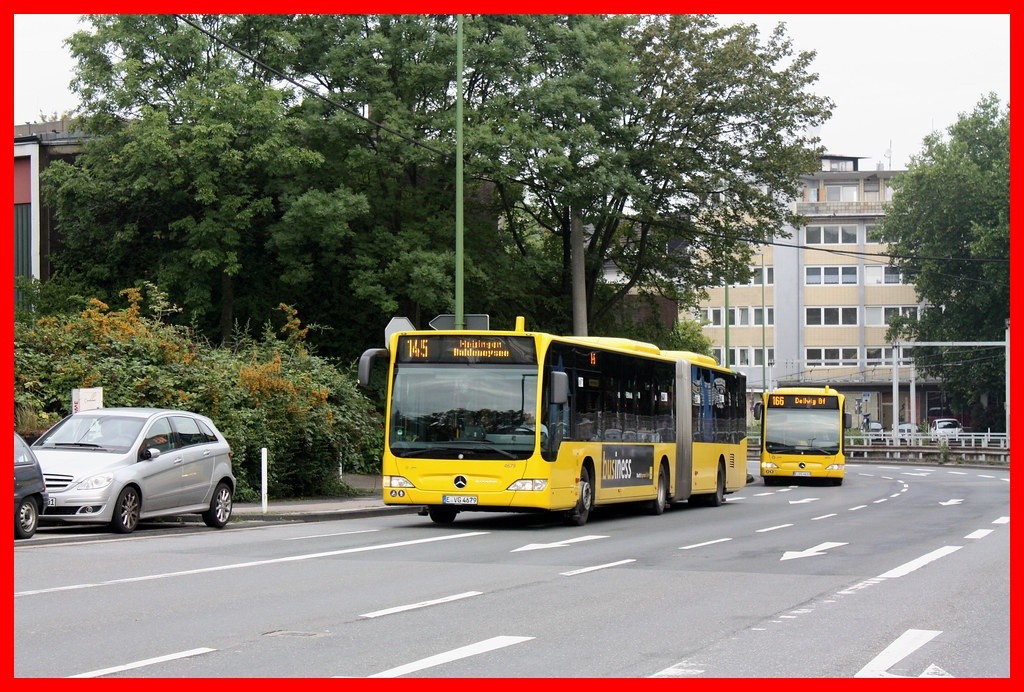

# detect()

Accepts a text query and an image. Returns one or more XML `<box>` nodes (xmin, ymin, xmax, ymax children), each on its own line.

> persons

<box><xmin>515</xmin><ymin>404</ymin><xmax>548</xmax><ymax>438</ymax></box>
<box><xmin>439</xmin><ymin>393</ymin><xmax>474</xmax><ymax>431</ymax></box>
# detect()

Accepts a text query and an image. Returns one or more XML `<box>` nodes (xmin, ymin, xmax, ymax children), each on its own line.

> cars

<box><xmin>29</xmin><ymin>406</ymin><xmax>237</xmax><ymax>534</ymax></box>
<box><xmin>892</xmin><ymin>423</ymin><xmax>920</xmax><ymax>442</ymax></box>
<box><xmin>929</xmin><ymin>418</ymin><xmax>964</xmax><ymax>444</ymax></box>
<box><xmin>868</xmin><ymin>422</ymin><xmax>886</xmax><ymax>441</ymax></box>
<box><xmin>14</xmin><ymin>431</ymin><xmax>50</xmax><ymax>539</ymax></box>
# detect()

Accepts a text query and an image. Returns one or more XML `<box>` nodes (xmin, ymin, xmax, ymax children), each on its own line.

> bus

<box><xmin>358</xmin><ymin>316</ymin><xmax>748</xmax><ymax>527</ymax></box>
<box><xmin>754</xmin><ymin>385</ymin><xmax>852</xmax><ymax>486</ymax></box>
